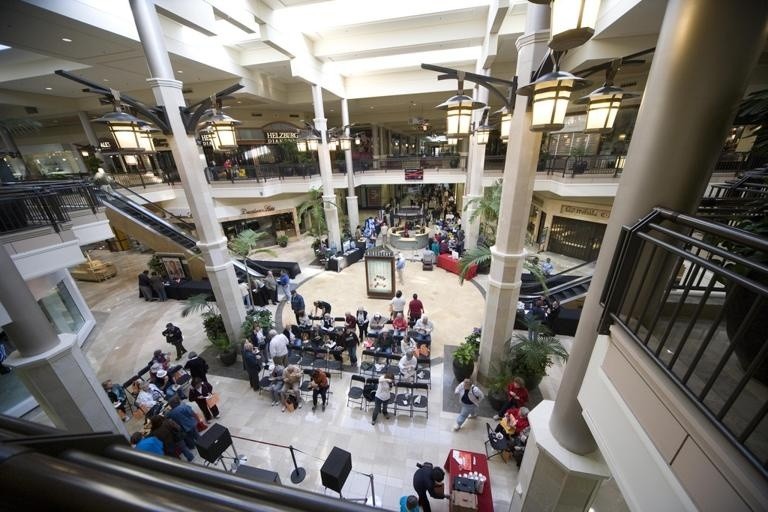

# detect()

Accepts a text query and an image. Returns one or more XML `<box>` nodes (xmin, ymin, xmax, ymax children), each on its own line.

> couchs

<box><xmin>70</xmin><ymin>260</ymin><xmax>117</xmax><ymax>282</ymax></box>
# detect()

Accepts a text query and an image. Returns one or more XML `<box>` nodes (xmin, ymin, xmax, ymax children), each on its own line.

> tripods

<box><xmin>214</xmin><ymin>456</ymin><xmax>247</xmax><ymax>473</ymax></box>
<box><xmin>336</xmin><ymin>491</ymin><xmax>367</xmax><ymax>502</ymax></box>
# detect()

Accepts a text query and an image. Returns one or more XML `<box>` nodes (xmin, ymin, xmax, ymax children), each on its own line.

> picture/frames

<box><xmin>160</xmin><ymin>256</ymin><xmax>187</xmax><ymax>281</ymax></box>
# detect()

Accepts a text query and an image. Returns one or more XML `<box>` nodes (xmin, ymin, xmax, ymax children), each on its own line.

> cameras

<box><xmin>388</xmin><ymin>381</ymin><xmax>394</xmax><ymax>390</ymax></box>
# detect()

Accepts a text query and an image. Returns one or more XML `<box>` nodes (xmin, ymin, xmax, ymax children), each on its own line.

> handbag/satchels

<box><xmin>206</xmin><ymin>393</ymin><xmax>220</xmax><ymax>409</ymax></box>
<box><xmin>260</xmin><ymin>376</ymin><xmax>271</xmax><ymax>387</ymax></box>
<box><xmin>430</xmin><ymin>481</ymin><xmax>444</xmax><ymax>498</ymax></box>
<box><xmin>145</xmin><ymin>404</ymin><xmax>162</xmax><ymax>420</ymax></box>
<box><xmin>285</xmin><ymin>395</ymin><xmax>298</xmax><ymax>412</ymax></box>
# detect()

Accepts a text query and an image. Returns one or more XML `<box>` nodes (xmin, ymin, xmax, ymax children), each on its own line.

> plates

<box><xmin>495</xmin><ymin>432</ymin><xmax>503</xmax><ymax>440</ymax></box>
<box><xmin>149</xmin><ymin>363</ymin><xmax>166</xmax><ymax>378</ymax></box>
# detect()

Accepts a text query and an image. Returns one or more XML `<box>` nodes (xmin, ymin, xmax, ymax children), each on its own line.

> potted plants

<box><xmin>279</xmin><ymin>138</ymin><xmax>316</xmax><ymax>176</ymax></box>
<box><xmin>277</xmin><ymin>234</ymin><xmax>288</xmax><ymax>247</ymax></box>
<box><xmin>488</xmin><ymin>313</ymin><xmax>569</xmax><ymax>412</ymax></box>
<box><xmin>452</xmin><ymin>328</ymin><xmax>481</xmax><ymax>383</ymax></box>
<box><xmin>214</xmin><ymin>331</ymin><xmax>238</xmax><ymax>366</ymax></box>
<box><xmin>296</xmin><ymin>186</ymin><xmax>342</xmax><ymax>262</ymax></box>
<box><xmin>571</xmin><ymin>146</ymin><xmax>588</xmax><ymax>174</ymax></box>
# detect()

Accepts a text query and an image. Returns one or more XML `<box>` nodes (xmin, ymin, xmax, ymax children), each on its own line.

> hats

<box><xmin>151</xmin><ymin>362</ymin><xmax>164</xmax><ymax>373</ymax></box>
<box><xmin>156</xmin><ymin>369</ymin><xmax>167</xmax><ymax>377</ymax></box>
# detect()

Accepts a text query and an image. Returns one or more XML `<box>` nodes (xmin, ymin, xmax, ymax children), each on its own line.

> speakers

<box><xmin>320</xmin><ymin>446</ymin><xmax>352</xmax><ymax>493</ymax></box>
<box><xmin>194</xmin><ymin>422</ymin><xmax>232</xmax><ymax>463</ymax></box>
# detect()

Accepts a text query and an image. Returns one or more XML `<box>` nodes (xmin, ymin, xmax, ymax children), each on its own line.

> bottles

<box><xmin>458</xmin><ymin>471</ymin><xmax>486</xmax><ymax>495</ymax></box>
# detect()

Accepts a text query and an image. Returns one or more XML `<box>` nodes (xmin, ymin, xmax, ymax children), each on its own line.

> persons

<box><xmin>311</xmin><ymin>183</ymin><xmax>466</xmax><ymax>285</ymax></box>
<box><xmin>94</xmin><ymin>167</ymin><xmax>116</xmax><ymax>202</ymax></box>
<box><xmin>240</xmin><ymin>269</ymin><xmax>434</xmax><ymax>425</ymax></box>
<box><xmin>528</xmin><ymin>255</ymin><xmax>562</xmax><ymax>343</ymax></box>
<box><xmin>138</xmin><ymin>269</ymin><xmax>157</xmax><ymax>302</ymax></box>
<box><xmin>210</xmin><ymin>156</ymin><xmax>233</xmax><ymax>181</ymax></box>
<box><xmin>400</xmin><ymin>494</ymin><xmax>423</xmax><ymax>510</ymax></box>
<box><xmin>493</xmin><ymin>377</ymin><xmax>530</xmax><ymax>466</ymax></box>
<box><xmin>101</xmin><ymin>321</ymin><xmax>220</xmax><ymax>463</ymax></box>
<box><xmin>453</xmin><ymin>377</ymin><xmax>484</xmax><ymax>431</ymax></box>
<box><xmin>414</xmin><ymin>462</ymin><xmax>450</xmax><ymax>512</ymax></box>
<box><xmin>150</xmin><ymin>270</ymin><xmax>169</xmax><ymax>302</ymax></box>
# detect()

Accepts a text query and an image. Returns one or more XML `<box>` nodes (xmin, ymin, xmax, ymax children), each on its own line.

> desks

<box><xmin>328</xmin><ymin>239</ymin><xmax>364</xmax><ymax>273</ymax></box>
<box><xmin>238</xmin><ymin>260</ymin><xmax>302</xmax><ymax>279</ymax></box>
<box><xmin>436</xmin><ymin>253</ymin><xmax>479</xmax><ymax>281</ymax></box>
<box><xmin>139</xmin><ymin>279</ymin><xmax>214</xmax><ymax>298</ymax></box>
<box><xmin>514</xmin><ymin>307</ymin><xmax>582</xmax><ymax>337</ymax></box>
<box><xmin>521</xmin><ymin>273</ymin><xmax>584</xmax><ymax>293</ymax></box>
<box><xmin>444</xmin><ymin>448</ymin><xmax>495</xmax><ymax>512</ymax></box>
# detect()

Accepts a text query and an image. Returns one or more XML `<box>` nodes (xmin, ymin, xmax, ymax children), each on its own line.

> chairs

<box><xmin>122</xmin><ymin>360</ymin><xmax>156</xmax><ymax>416</ymax></box>
<box><xmin>484</xmin><ymin>422</ymin><xmax>507</xmax><ymax>464</ymax></box>
<box><xmin>347</xmin><ymin>318</ymin><xmax>431</xmax><ymax>419</ymax></box>
<box><xmin>258</xmin><ymin>315</ymin><xmax>347</xmax><ymax>405</ymax></box>
